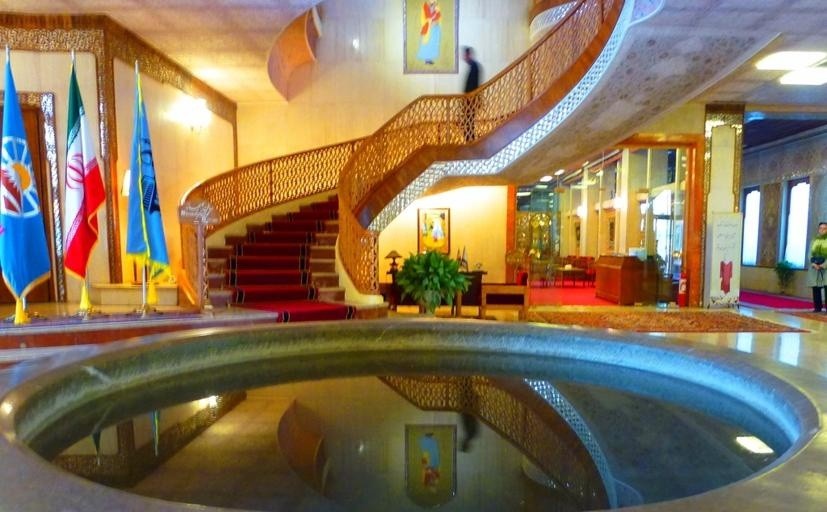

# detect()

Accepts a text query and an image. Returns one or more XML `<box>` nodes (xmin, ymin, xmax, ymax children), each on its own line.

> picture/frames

<box><xmin>417</xmin><ymin>207</ymin><xmax>451</xmax><ymax>256</ymax></box>
<box><xmin>792</xmin><ymin>311</ymin><xmax>827</xmax><ymax>321</ymax></box>
<box><xmin>403</xmin><ymin>0</ymin><xmax>459</xmax><ymax>74</ymax></box>
<box><xmin>404</xmin><ymin>423</ymin><xmax>458</xmax><ymax>508</ymax></box>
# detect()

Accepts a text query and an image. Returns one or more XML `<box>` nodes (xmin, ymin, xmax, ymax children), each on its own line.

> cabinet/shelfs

<box><xmin>595</xmin><ymin>254</ymin><xmax>643</xmax><ymax>305</ymax></box>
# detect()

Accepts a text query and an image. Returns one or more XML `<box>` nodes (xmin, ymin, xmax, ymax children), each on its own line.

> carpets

<box><xmin>530</xmin><ymin>312</ymin><xmax>810</xmax><ymax>332</ymax></box>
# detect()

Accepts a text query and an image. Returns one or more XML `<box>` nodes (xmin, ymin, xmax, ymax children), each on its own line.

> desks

<box><xmin>386</xmin><ymin>271</ymin><xmax>487</xmax><ymax>318</ymax></box>
<box><xmin>386</xmin><ymin>250</ymin><xmax>402</xmax><ymax>270</ymax></box>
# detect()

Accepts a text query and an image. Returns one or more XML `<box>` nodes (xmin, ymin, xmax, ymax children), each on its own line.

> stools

<box><xmin>478</xmin><ymin>282</ymin><xmax>529</xmax><ymax>319</ymax></box>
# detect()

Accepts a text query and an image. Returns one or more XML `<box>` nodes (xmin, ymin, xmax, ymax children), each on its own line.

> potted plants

<box><xmin>774</xmin><ymin>259</ymin><xmax>795</xmax><ymax>295</ymax></box>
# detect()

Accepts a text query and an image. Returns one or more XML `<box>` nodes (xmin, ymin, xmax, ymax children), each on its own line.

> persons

<box><xmin>806</xmin><ymin>221</ymin><xmax>827</xmax><ymax>312</ymax></box>
<box><xmin>455</xmin><ymin>375</ymin><xmax>482</xmax><ymax>453</ymax></box>
<box><xmin>419</xmin><ymin>432</ymin><xmax>441</xmax><ymax>491</ymax></box>
<box><xmin>456</xmin><ymin>46</ymin><xmax>482</xmax><ymax>142</ymax></box>
<box><xmin>416</xmin><ymin>1</ymin><xmax>441</xmax><ymax>65</ymax></box>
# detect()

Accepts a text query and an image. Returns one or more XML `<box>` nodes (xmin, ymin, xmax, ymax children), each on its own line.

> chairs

<box><xmin>524</xmin><ymin>255</ymin><xmax>594</xmax><ymax>288</ymax></box>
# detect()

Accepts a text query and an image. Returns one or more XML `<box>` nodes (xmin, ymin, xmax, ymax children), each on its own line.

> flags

<box><xmin>0</xmin><ymin>43</ymin><xmax>52</xmax><ymax>302</ymax></box>
<box><xmin>125</xmin><ymin>60</ymin><xmax>171</xmax><ymax>283</ymax></box>
<box><xmin>61</xmin><ymin>48</ymin><xmax>107</xmax><ymax>280</ymax></box>
